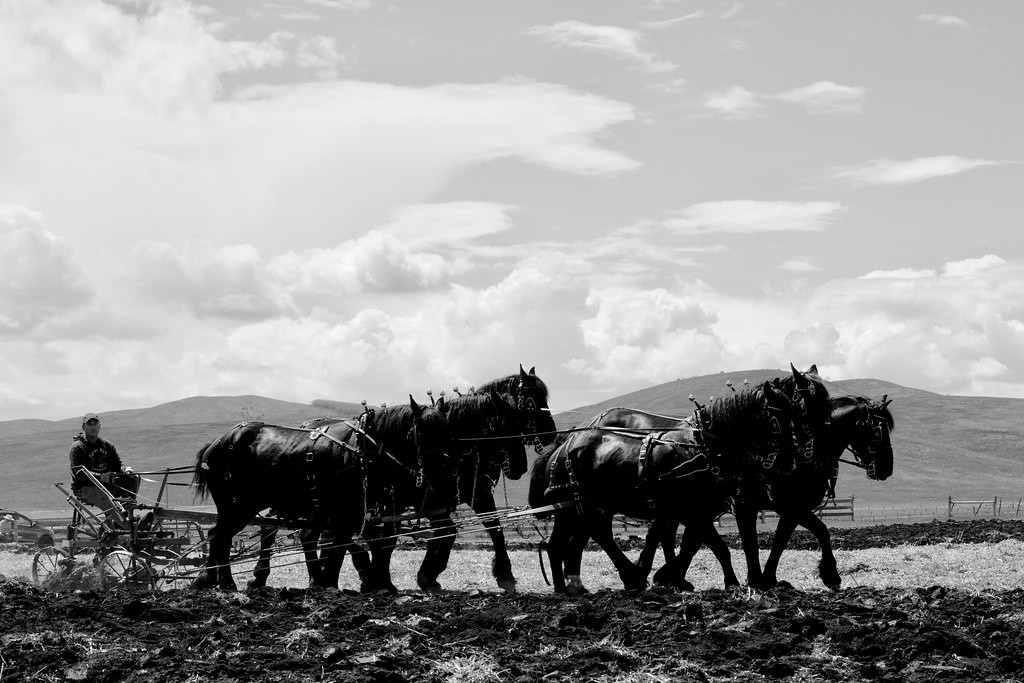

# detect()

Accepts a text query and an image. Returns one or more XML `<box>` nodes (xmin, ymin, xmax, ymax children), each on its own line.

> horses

<box><xmin>529</xmin><ymin>363</ymin><xmax>895</xmax><ymax>598</ymax></box>
<box><xmin>189</xmin><ymin>363</ymin><xmax>558</xmax><ymax>591</ymax></box>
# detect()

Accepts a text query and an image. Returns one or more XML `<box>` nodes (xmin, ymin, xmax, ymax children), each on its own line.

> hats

<box><xmin>83</xmin><ymin>413</ymin><xmax>100</xmax><ymax>424</ymax></box>
<box><xmin>3</xmin><ymin>515</ymin><xmax>14</xmax><ymax>521</ymax></box>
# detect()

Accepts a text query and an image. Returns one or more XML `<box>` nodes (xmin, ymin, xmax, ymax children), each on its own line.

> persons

<box><xmin>68</xmin><ymin>413</ymin><xmax>153</xmax><ymax>531</ymax></box>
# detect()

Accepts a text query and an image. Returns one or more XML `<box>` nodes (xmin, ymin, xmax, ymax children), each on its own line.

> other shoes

<box><xmin>138</xmin><ymin>512</ymin><xmax>153</xmax><ymax>529</ymax></box>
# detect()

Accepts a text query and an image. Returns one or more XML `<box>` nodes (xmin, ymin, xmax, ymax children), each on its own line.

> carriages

<box><xmin>32</xmin><ymin>363</ymin><xmax>895</xmax><ymax>591</ymax></box>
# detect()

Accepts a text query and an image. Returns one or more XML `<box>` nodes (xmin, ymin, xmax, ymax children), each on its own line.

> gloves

<box><xmin>124</xmin><ymin>468</ymin><xmax>134</xmax><ymax>479</ymax></box>
<box><xmin>101</xmin><ymin>472</ymin><xmax>116</xmax><ymax>484</ymax></box>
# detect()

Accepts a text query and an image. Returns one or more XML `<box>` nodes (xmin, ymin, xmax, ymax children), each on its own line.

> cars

<box><xmin>0</xmin><ymin>509</ymin><xmax>54</xmax><ymax>549</ymax></box>
